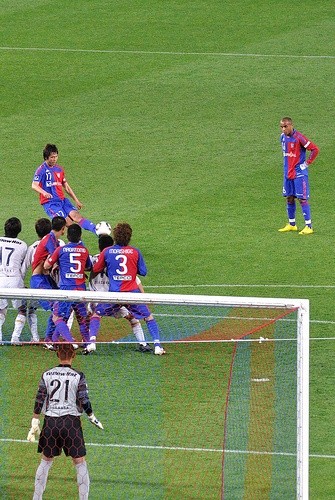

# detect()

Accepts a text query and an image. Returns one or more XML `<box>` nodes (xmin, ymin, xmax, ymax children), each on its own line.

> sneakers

<box><xmin>278</xmin><ymin>223</ymin><xmax>297</xmax><ymax>231</ymax></box>
<box><xmin>139</xmin><ymin>343</ymin><xmax>154</xmax><ymax>352</ymax></box>
<box><xmin>0</xmin><ymin>340</ymin><xmax>4</xmax><ymax>345</ymax></box>
<box><xmin>80</xmin><ymin>344</ymin><xmax>88</xmax><ymax>348</ymax></box>
<box><xmin>11</xmin><ymin>338</ymin><xmax>22</xmax><ymax>345</ymax></box>
<box><xmin>299</xmin><ymin>226</ymin><xmax>313</xmax><ymax>234</ymax></box>
<box><xmin>155</xmin><ymin>346</ymin><xmax>166</xmax><ymax>354</ymax></box>
<box><xmin>31</xmin><ymin>338</ymin><xmax>39</xmax><ymax>342</ymax></box>
<box><xmin>72</xmin><ymin>344</ymin><xmax>78</xmax><ymax>349</ymax></box>
<box><xmin>82</xmin><ymin>343</ymin><xmax>96</xmax><ymax>355</ymax></box>
<box><xmin>44</xmin><ymin>339</ymin><xmax>58</xmax><ymax>349</ymax></box>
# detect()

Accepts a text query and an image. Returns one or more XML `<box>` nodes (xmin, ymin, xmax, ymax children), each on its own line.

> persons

<box><xmin>26</xmin><ymin>344</ymin><xmax>104</xmax><ymax>500</ymax></box>
<box><xmin>32</xmin><ymin>143</ymin><xmax>95</xmax><ymax>239</ymax></box>
<box><xmin>1</xmin><ymin>217</ymin><xmax>167</xmax><ymax>356</ymax></box>
<box><xmin>278</xmin><ymin>117</ymin><xmax>320</xmax><ymax>236</ymax></box>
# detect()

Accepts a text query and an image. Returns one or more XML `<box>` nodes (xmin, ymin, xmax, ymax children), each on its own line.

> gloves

<box><xmin>27</xmin><ymin>418</ymin><xmax>41</xmax><ymax>442</ymax></box>
<box><xmin>88</xmin><ymin>413</ymin><xmax>104</xmax><ymax>429</ymax></box>
<box><xmin>295</xmin><ymin>161</ymin><xmax>308</xmax><ymax>173</ymax></box>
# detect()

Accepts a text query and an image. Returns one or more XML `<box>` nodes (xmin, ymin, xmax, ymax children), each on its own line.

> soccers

<box><xmin>95</xmin><ymin>221</ymin><xmax>111</xmax><ymax>237</ymax></box>
